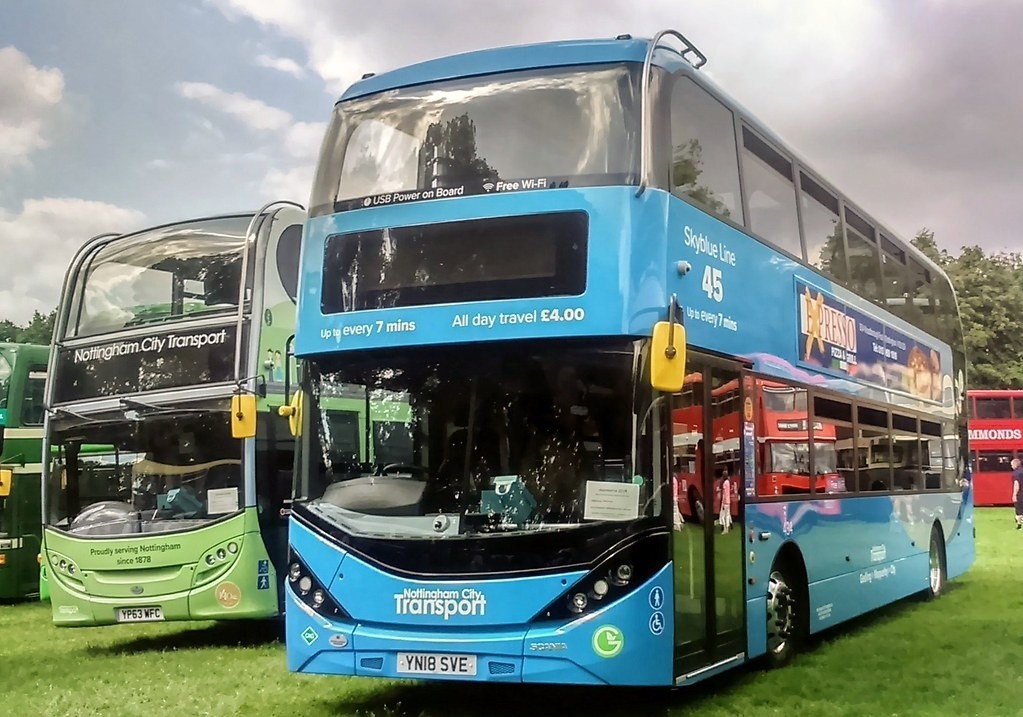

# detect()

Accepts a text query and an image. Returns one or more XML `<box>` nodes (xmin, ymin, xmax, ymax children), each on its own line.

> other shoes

<box><xmin>721</xmin><ymin>532</ymin><xmax>726</xmax><ymax>535</ymax></box>
<box><xmin>1016</xmin><ymin>524</ymin><xmax>1022</xmax><ymax>530</ymax></box>
<box><xmin>1016</xmin><ymin>520</ymin><xmax>1017</xmax><ymax>523</ymax></box>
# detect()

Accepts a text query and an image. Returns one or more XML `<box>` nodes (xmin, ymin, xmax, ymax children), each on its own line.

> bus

<box><xmin>837</xmin><ymin>436</ymin><xmax>962</xmax><ymax>491</ymax></box>
<box><xmin>0</xmin><ymin>342</ymin><xmax>147</xmax><ymax>600</ymax></box>
<box><xmin>279</xmin><ymin>28</ymin><xmax>973</xmax><ymax>695</ymax></box>
<box><xmin>967</xmin><ymin>389</ymin><xmax>1023</xmax><ymax>505</ymax></box>
<box><xmin>673</xmin><ymin>374</ymin><xmax>839</xmax><ymax>520</ymax></box>
<box><xmin>38</xmin><ymin>202</ymin><xmax>412</xmax><ymax>627</ymax></box>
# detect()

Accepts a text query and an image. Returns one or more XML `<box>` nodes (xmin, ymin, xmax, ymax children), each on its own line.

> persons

<box><xmin>719</xmin><ymin>470</ymin><xmax>732</xmax><ymax>536</ymax></box>
<box><xmin>1011</xmin><ymin>459</ymin><xmax>1023</xmax><ymax>530</ymax></box>
<box><xmin>673</xmin><ymin>472</ymin><xmax>685</xmax><ymax>532</ymax></box>
